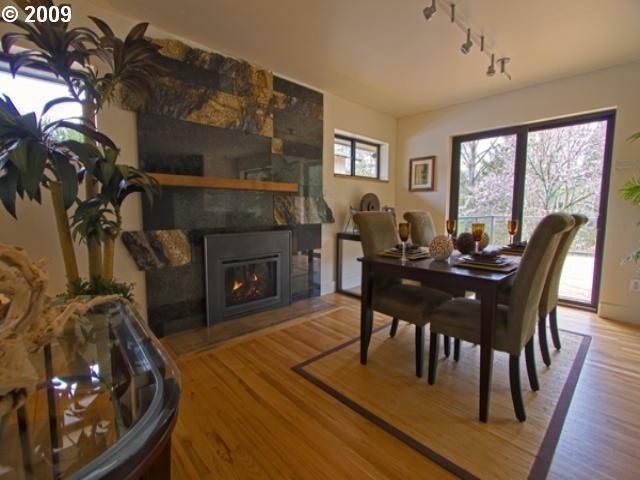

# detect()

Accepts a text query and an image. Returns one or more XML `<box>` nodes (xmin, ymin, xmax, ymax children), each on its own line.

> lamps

<box><xmin>423</xmin><ymin>0</ymin><xmax>473</xmax><ymax>56</ymax></box>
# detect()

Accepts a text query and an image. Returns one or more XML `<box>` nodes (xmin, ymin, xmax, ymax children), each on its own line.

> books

<box><xmin>499</xmin><ymin>240</ymin><xmax>528</xmax><ymax>257</ymax></box>
<box><xmin>453</xmin><ymin>246</ymin><xmax>518</xmax><ymax>275</ymax></box>
<box><xmin>377</xmin><ymin>242</ymin><xmax>432</xmax><ymax>261</ymax></box>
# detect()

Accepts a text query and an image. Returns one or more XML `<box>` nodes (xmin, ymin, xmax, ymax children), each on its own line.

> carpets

<box><xmin>290</xmin><ymin>318</ymin><xmax>593</xmax><ymax>479</ymax></box>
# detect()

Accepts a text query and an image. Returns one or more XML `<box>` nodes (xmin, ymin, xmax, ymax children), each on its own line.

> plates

<box><xmin>375</xmin><ymin>238</ymin><xmax>528</xmax><ymax>273</ymax></box>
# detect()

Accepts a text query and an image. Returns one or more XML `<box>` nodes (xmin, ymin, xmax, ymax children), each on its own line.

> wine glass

<box><xmin>398</xmin><ymin>223</ymin><xmax>409</xmax><ymax>262</ymax></box>
<box><xmin>472</xmin><ymin>222</ymin><xmax>485</xmax><ymax>253</ymax></box>
<box><xmin>508</xmin><ymin>218</ymin><xmax>520</xmax><ymax>245</ymax></box>
<box><xmin>446</xmin><ymin>219</ymin><xmax>457</xmax><ymax>240</ymax></box>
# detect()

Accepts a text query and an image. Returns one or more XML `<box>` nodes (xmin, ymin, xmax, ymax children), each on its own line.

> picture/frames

<box><xmin>409</xmin><ymin>156</ymin><xmax>435</xmax><ymax>191</ymax></box>
